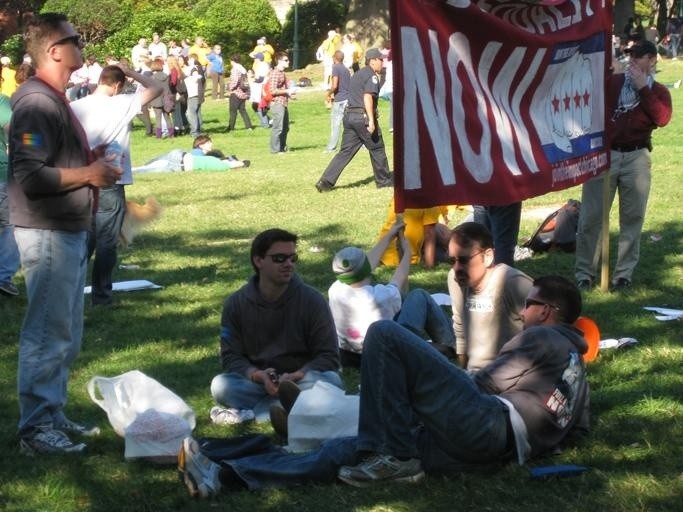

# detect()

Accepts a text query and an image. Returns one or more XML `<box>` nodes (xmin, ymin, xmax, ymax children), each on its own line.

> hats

<box><xmin>623</xmin><ymin>40</ymin><xmax>657</xmax><ymax>57</ymax></box>
<box><xmin>328</xmin><ymin>30</ymin><xmax>339</xmax><ymax>38</ymax></box>
<box><xmin>365</xmin><ymin>47</ymin><xmax>387</xmax><ymax>59</ymax></box>
<box><xmin>254</xmin><ymin>53</ymin><xmax>264</xmax><ymax>61</ymax></box>
<box><xmin>331</xmin><ymin>247</ymin><xmax>372</xmax><ymax>285</ymax></box>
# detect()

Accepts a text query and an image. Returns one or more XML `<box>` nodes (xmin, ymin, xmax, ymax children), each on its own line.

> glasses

<box><xmin>525</xmin><ymin>298</ymin><xmax>561</xmax><ymax>311</ymax></box>
<box><xmin>266</xmin><ymin>254</ymin><xmax>298</xmax><ymax>263</ymax></box>
<box><xmin>447</xmin><ymin>251</ymin><xmax>486</xmax><ymax>265</ymax></box>
<box><xmin>47</xmin><ymin>33</ymin><xmax>85</xmax><ymax>53</ymax></box>
<box><xmin>283</xmin><ymin>59</ymin><xmax>288</xmax><ymax>62</ymax></box>
<box><xmin>629</xmin><ymin>53</ymin><xmax>649</xmax><ymax>59</ymax></box>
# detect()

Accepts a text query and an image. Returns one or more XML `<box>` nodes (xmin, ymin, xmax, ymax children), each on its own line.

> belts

<box><xmin>347</xmin><ymin>108</ymin><xmax>365</xmax><ymax>113</ymax></box>
<box><xmin>181</xmin><ymin>152</ymin><xmax>186</xmax><ymax>172</ymax></box>
<box><xmin>611</xmin><ymin>144</ymin><xmax>645</xmax><ymax>151</ymax></box>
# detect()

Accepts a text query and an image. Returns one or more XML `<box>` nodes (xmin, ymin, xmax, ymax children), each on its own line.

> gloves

<box><xmin>226</xmin><ymin>155</ymin><xmax>237</xmax><ymax>160</ymax></box>
<box><xmin>244</xmin><ymin>160</ymin><xmax>251</xmax><ymax>168</ymax></box>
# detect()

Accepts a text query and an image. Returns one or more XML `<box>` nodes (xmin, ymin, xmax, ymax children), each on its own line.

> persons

<box><xmin>208</xmin><ymin>225</ymin><xmax>346</xmax><ymax>427</ymax></box>
<box><xmin>268</xmin><ymin>221</ymin><xmax>534</xmax><ymax>445</ymax></box>
<box><xmin>2</xmin><ymin>29</ymin><xmax>403</xmax><ymax>307</ymax></box>
<box><xmin>174</xmin><ymin>275</ymin><xmax>598</xmax><ymax>503</ymax></box>
<box><xmin>468</xmin><ymin>202</ymin><xmax>524</xmax><ymax>268</ymax></box>
<box><xmin>610</xmin><ymin>1</ymin><xmax>683</xmax><ymax>71</ymax></box>
<box><xmin>377</xmin><ymin>205</ymin><xmax>463</xmax><ymax>272</ymax></box>
<box><xmin>573</xmin><ymin>41</ymin><xmax>675</xmax><ymax>289</ymax></box>
<box><xmin>0</xmin><ymin>9</ymin><xmax>125</xmax><ymax>460</ymax></box>
<box><xmin>328</xmin><ymin>216</ymin><xmax>460</xmax><ymax>367</ymax></box>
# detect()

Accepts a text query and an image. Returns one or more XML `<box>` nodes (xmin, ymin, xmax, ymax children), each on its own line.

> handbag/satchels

<box><xmin>163</xmin><ymin>91</ymin><xmax>175</xmax><ymax>112</ymax></box>
<box><xmin>240</xmin><ymin>73</ymin><xmax>250</xmax><ymax>91</ymax></box>
<box><xmin>175</xmin><ymin>75</ymin><xmax>187</xmax><ymax>93</ymax></box>
<box><xmin>260</xmin><ymin>83</ymin><xmax>272</xmax><ymax>102</ymax></box>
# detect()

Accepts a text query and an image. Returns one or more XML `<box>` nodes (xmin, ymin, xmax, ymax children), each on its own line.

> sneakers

<box><xmin>0</xmin><ymin>280</ymin><xmax>20</xmax><ymax>296</ymax></box>
<box><xmin>178</xmin><ymin>435</ymin><xmax>222</xmax><ymax>502</ymax></box>
<box><xmin>268</xmin><ymin>406</ymin><xmax>289</xmax><ymax>445</ymax></box>
<box><xmin>323</xmin><ymin>146</ymin><xmax>338</xmax><ymax>153</ymax></box>
<box><xmin>277</xmin><ymin>380</ymin><xmax>301</xmax><ymax>413</ymax></box>
<box><xmin>53</xmin><ymin>419</ymin><xmax>100</xmax><ymax>442</ymax></box>
<box><xmin>181</xmin><ymin>126</ymin><xmax>187</xmax><ymax>135</ymax></box>
<box><xmin>174</xmin><ymin>128</ymin><xmax>180</xmax><ymax>136</ymax></box>
<box><xmin>339</xmin><ymin>454</ymin><xmax>426</xmax><ymax>488</ymax></box>
<box><xmin>19</xmin><ymin>423</ymin><xmax>87</xmax><ymax>459</ymax></box>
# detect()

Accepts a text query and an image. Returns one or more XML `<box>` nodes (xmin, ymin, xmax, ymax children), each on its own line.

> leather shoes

<box><xmin>316</xmin><ymin>180</ymin><xmax>335</xmax><ymax>192</ymax></box>
<box><xmin>611</xmin><ymin>279</ymin><xmax>632</xmax><ymax>293</ymax></box>
<box><xmin>577</xmin><ymin>276</ymin><xmax>597</xmax><ymax>291</ymax></box>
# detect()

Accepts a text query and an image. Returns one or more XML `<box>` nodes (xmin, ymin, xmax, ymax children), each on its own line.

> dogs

<box><xmin>117</xmin><ymin>196</ymin><xmax>163</xmax><ymax>251</ymax></box>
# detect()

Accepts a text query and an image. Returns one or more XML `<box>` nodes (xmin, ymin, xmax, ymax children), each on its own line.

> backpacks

<box><xmin>315</xmin><ymin>46</ymin><xmax>324</xmax><ymax>62</ymax></box>
<box><xmin>520</xmin><ymin>199</ymin><xmax>580</xmax><ymax>253</ymax></box>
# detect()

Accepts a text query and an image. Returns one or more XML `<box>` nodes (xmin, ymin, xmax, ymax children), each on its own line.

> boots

<box><xmin>155</xmin><ymin>128</ymin><xmax>162</xmax><ymax>138</ymax></box>
<box><xmin>166</xmin><ymin>128</ymin><xmax>174</xmax><ymax>137</ymax></box>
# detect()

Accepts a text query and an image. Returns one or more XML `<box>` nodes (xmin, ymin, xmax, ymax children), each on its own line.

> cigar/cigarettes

<box><xmin>630</xmin><ymin>66</ymin><xmax>634</xmax><ymax>71</ymax></box>
